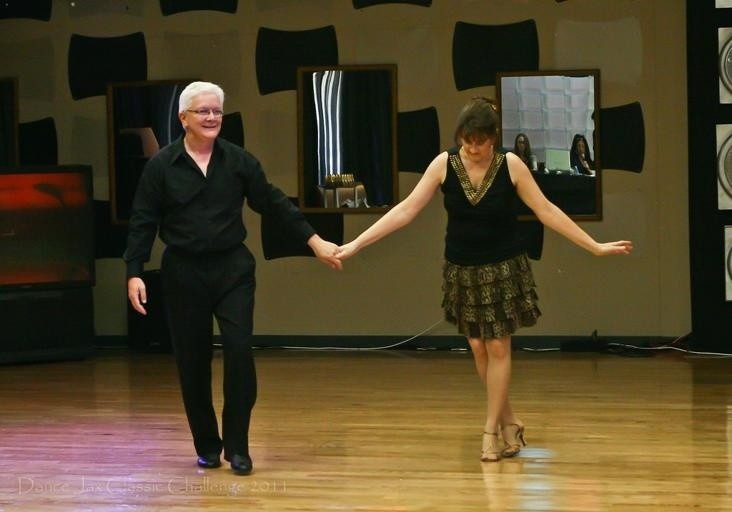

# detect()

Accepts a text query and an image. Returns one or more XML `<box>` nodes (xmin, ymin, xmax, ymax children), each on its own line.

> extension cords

<box><xmin>559</xmin><ymin>340</ymin><xmax>608</xmax><ymax>353</ymax></box>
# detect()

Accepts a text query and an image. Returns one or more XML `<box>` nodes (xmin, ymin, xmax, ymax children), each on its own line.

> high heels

<box><xmin>482</xmin><ymin>430</ymin><xmax>500</xmax><ymax>462</ymax></box>
<box><xmin>501</xmin><ymin>421</ymin><xmax>526</xmax><ymax>456</ymax></box>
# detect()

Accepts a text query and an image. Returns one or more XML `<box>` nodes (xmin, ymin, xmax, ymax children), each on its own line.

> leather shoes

<box><xmin>197</xmin><ymin>440</ymin><xmax>223</xmax><ymax>467</ymax></box>
<box><xmin>223</xmin><ymin>451</ymin><xmax>252</xmax><ymax>474</ymax></box>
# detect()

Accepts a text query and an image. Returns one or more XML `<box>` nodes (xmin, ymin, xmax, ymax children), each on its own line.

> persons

<box><xmin>512</xmin><ymin>133</ymin><xmax>537</xmax><ymax>171</ymax></box>
<box><xmin>570</xmin><ymin>134</ymin><xmax>595</xmax><ymax>176</ymax></box>
<box><xmin>123</xmin><ymin>80</ymin><xmax>343</xmax><ymax>474</ymax></box>
<box><xmin>338</xmin><ymin>96</ymin><xmax>633</xmax><ymax>462</ymax></box>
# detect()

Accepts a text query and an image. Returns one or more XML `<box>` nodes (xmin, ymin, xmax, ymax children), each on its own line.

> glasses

<box><xmin>189</xmin><ymin>109</ymin><xmax>223</xmax><ymax>117</ymax></box>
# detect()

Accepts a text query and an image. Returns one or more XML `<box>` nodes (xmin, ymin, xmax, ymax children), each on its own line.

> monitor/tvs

<box><xmin>0</xmin><ymin>165</ymin><xmax>96</xmax><ymax>291</ymax></box>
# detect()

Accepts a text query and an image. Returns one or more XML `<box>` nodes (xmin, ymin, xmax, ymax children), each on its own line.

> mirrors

<box><xmin>494</xmin><ymin>68</ymin><xmax>604</xmax><ymax>223</ymax></box>
<box><xmin>295</xmin><ymin>63</ymin><xmax>401</xmax><ymax>217</ymax></box>
<box><xmin>104</xmin><ymin>77</ymin><xmax>205</xmax><ymax>227</ymax></box>
